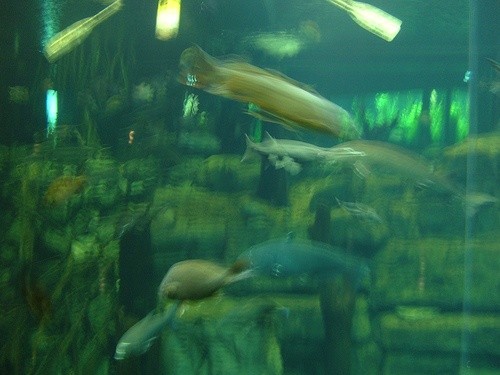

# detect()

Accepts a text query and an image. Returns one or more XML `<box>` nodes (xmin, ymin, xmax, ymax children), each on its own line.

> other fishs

<box><xmin>42</xmin><ymin>0</ymin><xmax>500</xmax><ymax>363</ymax></box>
